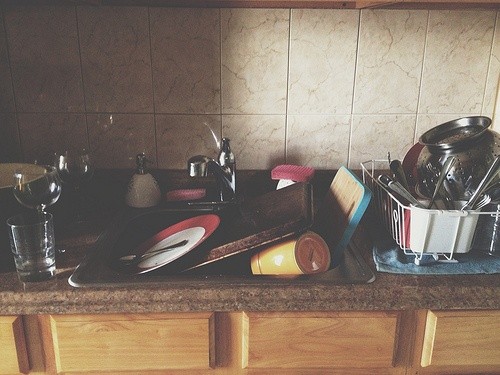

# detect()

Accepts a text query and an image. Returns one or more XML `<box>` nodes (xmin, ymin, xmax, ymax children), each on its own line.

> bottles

<box><xmin>216</xmin><ymin>136</ymin><xmax>236</xmax><ymax>204</ymax></box>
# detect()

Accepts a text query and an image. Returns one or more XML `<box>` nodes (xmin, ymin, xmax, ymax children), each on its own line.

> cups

<box><xmin>13</xmin><ymin>166</ymin><xmax>61</xmax><ymax>212</ymax></box>
<box><xmin>7</xmin><ymin>213</ymin><xmax>57</xmax><ymax>283</ymax></box>
<box><xmin>251</xmin><ymin>233</ymin><xmax>331</xmax><ymax>275</ymax></box>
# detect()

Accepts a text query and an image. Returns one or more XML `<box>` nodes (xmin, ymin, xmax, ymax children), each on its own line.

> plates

<box><xmin>136</xmin><ymin>213</ymin><xmax>221</xmax><ymax>279</ymax></box>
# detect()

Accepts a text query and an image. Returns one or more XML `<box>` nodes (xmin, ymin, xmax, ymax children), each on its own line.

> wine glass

<box><xmin>54</xmin><ymin>146</ymin><xmax>100</xmax><ymax>228</ymax></box>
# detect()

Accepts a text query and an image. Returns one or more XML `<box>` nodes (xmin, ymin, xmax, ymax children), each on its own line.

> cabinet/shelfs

<box><xmin>0</xmin><ymin>311</ymin><xmax>500</xmax><ymax>375</ymax></box>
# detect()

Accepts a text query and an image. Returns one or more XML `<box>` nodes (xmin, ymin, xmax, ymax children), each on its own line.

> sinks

<box><xmin>67</xmin><ymin>203</ymin><xmax>375</xmax><ymax>288</ymax></box>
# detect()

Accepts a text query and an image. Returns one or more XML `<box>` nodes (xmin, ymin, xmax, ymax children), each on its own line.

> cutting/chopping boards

<box><xmin>316</xmin><ymin>167</ymin><xmax>373</xmax><ymax>267</ymax></box>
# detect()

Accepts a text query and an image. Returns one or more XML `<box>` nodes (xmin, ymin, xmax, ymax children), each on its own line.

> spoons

<box><xmin>116</xmin><ymin>239</ymin><xmax>188</xmax><ymax>268</ymax></box>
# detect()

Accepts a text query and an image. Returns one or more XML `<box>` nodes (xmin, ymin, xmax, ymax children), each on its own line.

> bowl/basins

<box><xmin>401</xmin><ymin>116</ymin><xmax>500</xmax><ymax>199</ymax></box>
<box><xmin>1</xmin><ymin>163</ymin><xmax>46</xmax><ymax>212</ymax></box>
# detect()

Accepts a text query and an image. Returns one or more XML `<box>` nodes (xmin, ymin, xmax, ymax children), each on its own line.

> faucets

<box><xmin>188</xmin><ymin>137</ymin><xmax>236</xmax><ymax>201</ymax></box>
